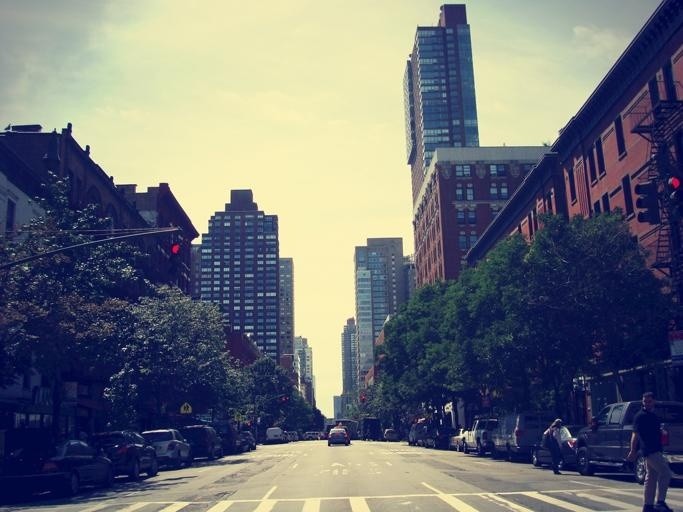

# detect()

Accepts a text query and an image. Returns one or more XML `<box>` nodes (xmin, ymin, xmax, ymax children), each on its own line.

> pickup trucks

<box><xmin>460</xmin><ymin>419</ymin><xmax>503</xmax><ymax>456</ymax></box>
<box><xmin>575</xmin><ymin>400</ymin><xmax>683</xmax><ymax>485</ymax></box>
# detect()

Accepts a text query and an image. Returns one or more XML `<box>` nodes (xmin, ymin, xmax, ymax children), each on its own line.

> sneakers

<box><xmin>554</xmin><ymin>471</ymin><xmax>561</xmax><ymax>474</ymax></box>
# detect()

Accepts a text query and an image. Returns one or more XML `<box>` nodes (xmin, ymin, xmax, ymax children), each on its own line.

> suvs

<box><xmin>86</xmin><ymin>424</ymin><xmax>257</xmax><ymax>481</ymax></box>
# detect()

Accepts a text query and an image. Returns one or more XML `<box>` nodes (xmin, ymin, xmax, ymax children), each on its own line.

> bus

<box><xmin>361</xmin><ymin>418</ymin><xmax>382</xmax><ymax>441</ymax></box>
<box><xmin>335</xmin><ymin>419</ymin><xmax>359</xmax><ymax>440</ymax></box>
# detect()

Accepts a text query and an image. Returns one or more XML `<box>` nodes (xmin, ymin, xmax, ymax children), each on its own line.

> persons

<box><xmin>547</xmin><ymin>416</ymin><xmax>562</xmax><ymax>476</ymax></box>
<box><xmin>627</xmin><ymin>390</ymin><xmax>674</xmax><ymax>511</ymax></box>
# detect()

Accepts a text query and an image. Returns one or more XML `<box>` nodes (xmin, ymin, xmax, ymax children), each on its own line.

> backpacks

<box><xmin>542</xmin><ymin>428</ymin><xmax>555</xmax><ymax>449</ymax></box>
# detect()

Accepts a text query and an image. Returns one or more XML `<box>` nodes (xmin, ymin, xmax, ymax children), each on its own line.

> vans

<box><xmin>491</xmin><ymin>410</ymin><xmax>560</xmax><ymax>463</ymax></box>
<box><xmin>305</xmin><ymin>431</ymin><xmax>319</xmax><ymax>440</ymax></box>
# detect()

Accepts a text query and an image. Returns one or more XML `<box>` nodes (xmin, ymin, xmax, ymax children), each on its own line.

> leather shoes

<box><xmin>643</xmin><ymin>504</ymin><xmax>673</xmax><ymax>512</ymax></box>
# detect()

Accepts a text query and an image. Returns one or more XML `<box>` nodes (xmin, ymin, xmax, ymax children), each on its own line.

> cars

<box><xmin>0</xmin><ymin>438</ymin><xmax>115</xmax><ymax>497</ymax></box>
<box><xmin>328</xmin><ymin>425</ymin><xmax>350</xmax><ymax>446</ymax></box>
<box><xmin>383</xmin><ymin>428</ymin><xmax>398</xmax><ymax>441</ymax></box>
<box><xmin>448</xmin><ymin>427</ymin><xmax>471</xmax><ymax>452</ymax></box>
<box><xmin>266</xmin><ymin>426</ymin><xmax>299</xmax><ymax>443</ymax></box>
<box><xmin>408</xmin><ymin>423</ymin><xmax>456</xmax><ymax>450</ymax></box>
<box><xmin>530</xmin><ymin>423</ymin><xmax>587</xmax><ymax>470</ymax></box>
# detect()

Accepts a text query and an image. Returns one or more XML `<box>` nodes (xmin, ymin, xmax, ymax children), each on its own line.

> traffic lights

<box><xmin>280</xmin><ymin>394</ymin><xmax>291</xmax><ymax>401</ymax></box>
<box><xmin>170</xmin><ymin>242</ymin><xmax>181</xmax><ymax>277</ymax></box>
<box><xmin>662</xmin><ymin>173</ymin><xmax>683</xmax><ymax>221</ymax></box>
<box><xmin>360</xmin><ymin>392</ymin><xmax>365</xmax><ymax>402</ymax></box>
<box><xmin>634</xmin><ymin>174</ymin><xmax>662</xmax><ymax>225</ymax></box>
